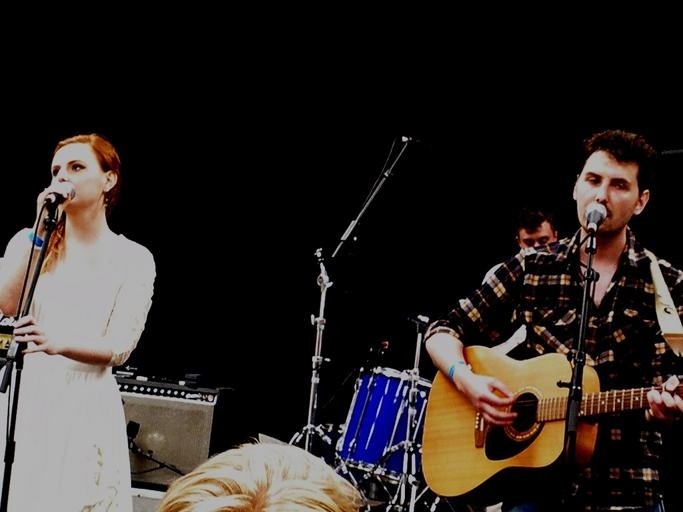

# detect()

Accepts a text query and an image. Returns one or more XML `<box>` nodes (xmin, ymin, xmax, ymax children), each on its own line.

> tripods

<box><xmin>289</xmin><ymin>137</ymin><xmax>449</xmax><ymax>512</ymax></box>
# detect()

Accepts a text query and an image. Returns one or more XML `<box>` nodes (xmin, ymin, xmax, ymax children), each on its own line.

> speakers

<box><xmin>113</xmin><ymin>364</ymin><xmax>233</xmax><ymax>492</ymax></box>
<box><xmin>0</xmin><ymin>309</ymin><xmax>16</xmax><ymax>372</ymax></box>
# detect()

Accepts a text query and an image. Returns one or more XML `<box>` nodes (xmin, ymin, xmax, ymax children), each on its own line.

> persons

<box><xmin>159</xmin><ymin>442</ymin><xmax>362</xmax><ymax>512</ymax></box>
<box><xmin>0</xmin><ymin>133</ymin><xmax>157</xmax><ymax>512</ymax></box>
<box><xmin>515</xmin><ymin>209</ymin><xmax>559</xmax><ymax>248</ymax></box>
<box><xmin>423</xmin><ymin>129</ymin><xmax>683</xmax><ymax>512</ymax></box>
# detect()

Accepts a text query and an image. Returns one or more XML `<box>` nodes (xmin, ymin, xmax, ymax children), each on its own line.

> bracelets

<box><xmin>448</xmin><ymin>361</ymin><xmax>467</xmax><ymax>380</ymax></box>
<box><xmin>27</xmin><ymin>230</ymin><xmax>44</xmax><ymax>248</ymax></box>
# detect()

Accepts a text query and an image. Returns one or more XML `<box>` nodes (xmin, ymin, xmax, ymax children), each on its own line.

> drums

<box><xmin>338</xmin><ymin>366</ymin><xmax>431</xmax><ymax>488</ymax></box>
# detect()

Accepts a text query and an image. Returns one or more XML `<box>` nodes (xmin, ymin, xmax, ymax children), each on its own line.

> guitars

<box><xmin>420</xmin><ymin>345</ymin><xmax>683</xmax><ymax>507</ymax></box>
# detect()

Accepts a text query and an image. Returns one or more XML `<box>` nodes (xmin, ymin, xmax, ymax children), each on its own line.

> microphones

<box><xmin>127</xmin><ymin>421</ymin><xmax>140</xmax><ymax>446</ymax></box>
<box><xmin>583</xmin><ymin>202</ymin><xmax>607</xmax><ymax>235</ymax></box>
<box><xmin>44</xmin><ymin>182</ymin><xmax>76</xmax><ymax>203</ymax></box>
